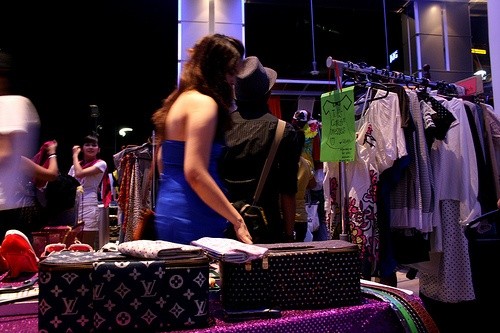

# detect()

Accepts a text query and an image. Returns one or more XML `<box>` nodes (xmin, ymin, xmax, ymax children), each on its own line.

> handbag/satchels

<box><xmin>228</xmin><ymin>201</ymin><xmax>270</xmax><ymax>244</ymax></box>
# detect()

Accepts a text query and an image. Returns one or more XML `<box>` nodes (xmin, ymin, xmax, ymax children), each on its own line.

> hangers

<box><xmin>123</xmin><ymin>136</ymin><xmax>152</xmax><ymax>162</ymax></box>
<box><xmin>341</xmin><ymin>61</ymin><xmax>458</xmax><ymax>107</ymax></box>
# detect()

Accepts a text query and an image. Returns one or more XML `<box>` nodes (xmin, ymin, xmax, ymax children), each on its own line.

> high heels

<box><xmin>1</xmin><ymin>229</ymin><xmax>39</xmax><ymax>280</ymax></box>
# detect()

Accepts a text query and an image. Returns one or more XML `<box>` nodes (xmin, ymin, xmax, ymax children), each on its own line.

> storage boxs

<box><xmin>220</xmin><ymin>239</ymin><xmax>361</xmax><ymax>312</ymax></box>
<box><xmin>37</xmin><ymin>250</ymin><xmax>210</xmax><ymax>333</ymax></box>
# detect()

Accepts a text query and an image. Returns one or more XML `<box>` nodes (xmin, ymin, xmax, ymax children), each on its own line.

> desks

<box><xmin>0</xmin><ymin>279</ymin><xmax>422</xmax><ymax>333</ymax></box>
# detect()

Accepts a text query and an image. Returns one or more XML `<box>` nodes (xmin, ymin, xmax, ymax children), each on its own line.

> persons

<box><xmin>0</xmin><ymin>33</ymin><xmax>317</xmax><ymax>243</ymax></box>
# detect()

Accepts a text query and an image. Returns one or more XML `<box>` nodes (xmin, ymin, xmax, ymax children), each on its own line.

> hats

<box><xmin>232</xmin><ymin>56</ymin><xmax>277</xmax><ymax>102</ymax></box>
